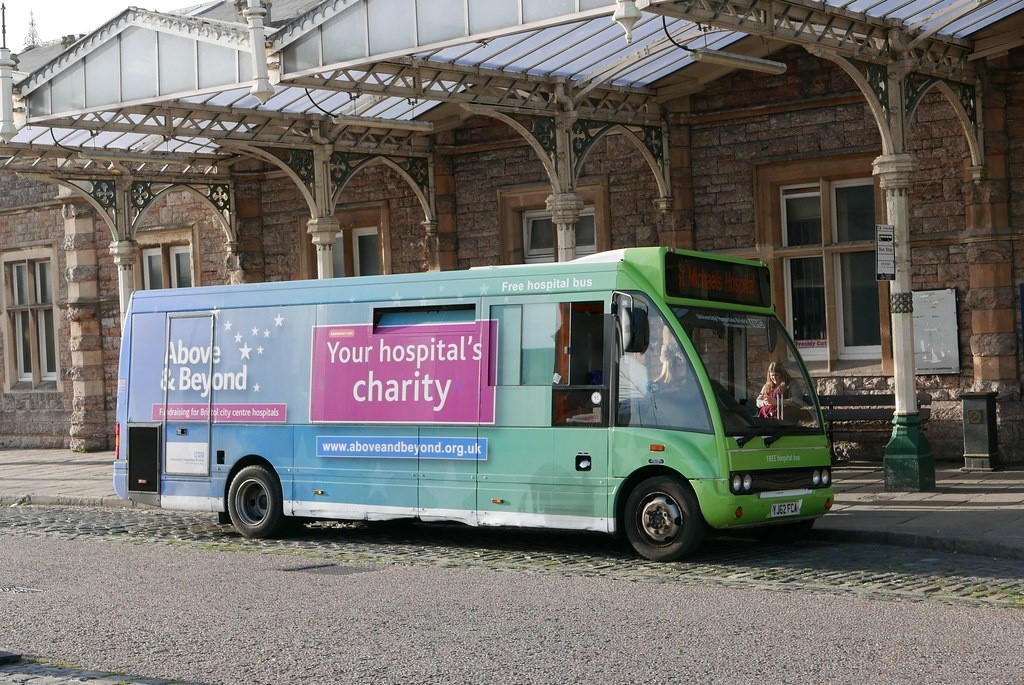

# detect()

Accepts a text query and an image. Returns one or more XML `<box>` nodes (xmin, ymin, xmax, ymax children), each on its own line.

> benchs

<box><xmin>801</xmin><ymin>394</ymin><xmax>932</xmax><ymax>442</ymax></box>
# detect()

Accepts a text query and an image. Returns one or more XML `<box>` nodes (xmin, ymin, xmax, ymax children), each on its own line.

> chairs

<box><xmin>584</xmin><ymin>371</ymin><xmax>603</xmax><ymax>410</ymax></box>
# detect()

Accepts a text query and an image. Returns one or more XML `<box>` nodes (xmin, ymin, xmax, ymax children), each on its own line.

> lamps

<box><xmin>689</xmin><ymin>27</ymin><xmax>787</xmax><ymax>75</ymax></box>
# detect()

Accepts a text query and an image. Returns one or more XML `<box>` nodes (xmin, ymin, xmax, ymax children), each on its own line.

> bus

<box><xmin>113</xmin><ymin>244</ymin><xmax>836</xmax><ymax>563</ymax></box>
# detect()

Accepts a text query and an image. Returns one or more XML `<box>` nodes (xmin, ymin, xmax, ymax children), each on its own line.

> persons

<box><xmin>757</xmin><ymin>361</ymin><xmax>804</xmax><ymax>420</ymax></box>
<box><xmin>618</xmin><ymin>352</ymin><xmax>653</xmax><ymax>418</ymax></box>
